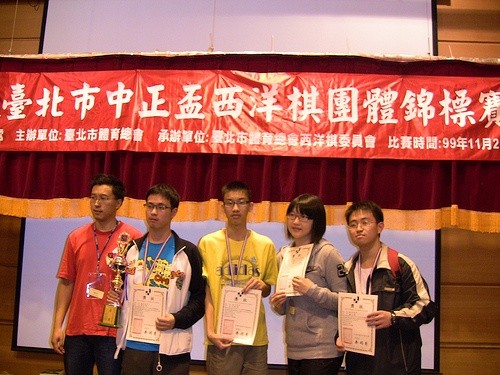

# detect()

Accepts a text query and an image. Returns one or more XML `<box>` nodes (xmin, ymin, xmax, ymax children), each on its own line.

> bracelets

<box><xmin>391</xmin><ymin>313</ymin><xmax>396</xmax><ymax>324</ymax></box>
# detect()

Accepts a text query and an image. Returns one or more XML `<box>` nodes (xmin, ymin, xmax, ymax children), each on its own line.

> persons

<box><xmin>197</xmin><ymin>182</ymin><xmax>279</xmax><ymax>375</ymax></box>
<box><xmin>51</xmin><ymin>176</ymin><xmax>144</xmax><ymax>375</ymax></box>
<box><xmin>270</xmin><ymin>194</ymin><xmax>348</xmax><ymax>375</ymax></box>
<box><xmin>108</xmin><ymin>184</ymin><xmax>206</xmax><ymax>375</ymax></box>
<box><xmin>334</xmin><ymin>201</ymin><xmax>436</xmax><ymax>375</ymax></box>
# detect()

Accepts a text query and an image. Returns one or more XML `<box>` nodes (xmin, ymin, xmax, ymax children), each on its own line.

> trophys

<box><xmin>98</xmin><ymin>232</ymin><xmax>130</xmax><ymax>328</ymax></box>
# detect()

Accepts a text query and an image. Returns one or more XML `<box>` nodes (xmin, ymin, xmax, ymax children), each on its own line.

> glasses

<box><xmin>142</xmin><ymin>203</ymin><xmax>171</xmax><ymax>211</ymax></box>
<box><xmin>222</xmin><ymin>199</ymin><xmax>250</xmax><ymax>208</ymax></box>
<box><xmin>348</xmin><ymin>220</ymin><xmax>377</xmax><ymax>229</ymax></box>
<box><xmin>89</xmin><ymin>196</ymin><xmax>115</xmax><ymax>202</ymax></box>
<box><xmin>287</xmin><ymin>212</ymin><xmax>313</xmax><ymax>223</ymax></box>
